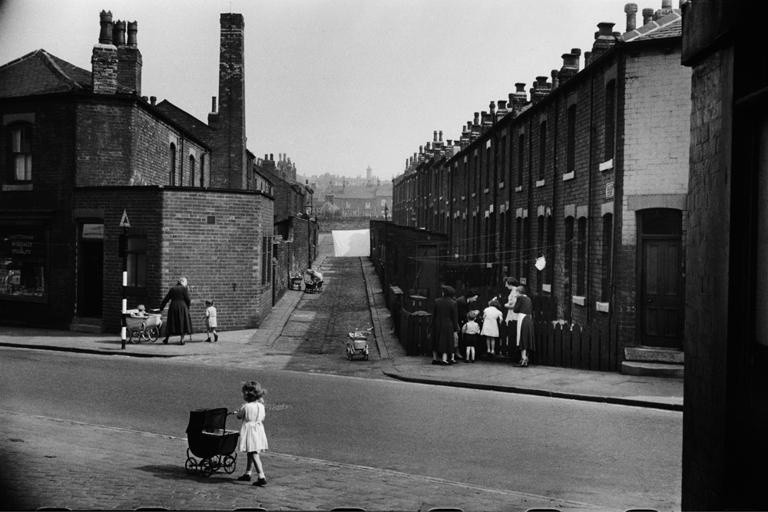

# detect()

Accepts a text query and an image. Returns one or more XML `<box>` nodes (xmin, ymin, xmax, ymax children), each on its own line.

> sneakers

<box><xmin>180</xmin><ymin>340</ymin><xmax>185</xmax><ymax>345</ymax></box>
<box><xmin>432</xmin><ymin>357</ymin><xmax>475</xmax><ymax>365</ymax></box>
<box><xmin>238</xmin><ymin>474</ymin><xmax>252</xmax><ymax>480</ymax></box>
<box><xmin>205</xmin><ymin>337</ymin><xmax>211</xmax><ymax>342</ymax></box>
<box><xmin>163</xmin><ymin>339</ymin><xmax>168</xmax><ymax>344</ymax></box>
<box><xmin>214</xmin><ymin>333</ymin><xmax>218</xmax><ymax>341</ymax></box>
<box><xmin>252</xmin><ymin>477</ymin><xmax>267</xmax><ymax>486</ymax></box>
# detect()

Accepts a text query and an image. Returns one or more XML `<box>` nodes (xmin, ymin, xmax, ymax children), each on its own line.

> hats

<box><xmin>440</xmin><ymin>285</ymin><xmax>455</xmax><ymax>296</ymax></box>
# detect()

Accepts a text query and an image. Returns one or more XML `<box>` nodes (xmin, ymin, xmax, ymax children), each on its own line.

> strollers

<box><xmin>184</xmin><ymin>407</ymin><xmax>241</xmax><ymax>478</ymax></box>
<box><xmin>126</xmin><ymin>308</ymin><xmax>163</xmax><ymax>344</ymax></box>
<box><xmin>345</xmin><ymin>331</ymin><xmax>369</xmax><ymax>361</ymax></box>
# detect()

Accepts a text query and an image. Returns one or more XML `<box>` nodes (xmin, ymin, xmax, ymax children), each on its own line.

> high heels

<box><xmin>518</xmin><ymin>357</ymin><xmax>528</xmax><ymax>366</ymax></box>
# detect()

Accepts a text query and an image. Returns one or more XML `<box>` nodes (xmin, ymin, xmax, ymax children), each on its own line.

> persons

<box><xmin>202</xmin><ymin>299</ymin><xmax>218</xmax><ymax>342</ymax></box>
<box><xmin>136</xmin><ymin>305</ymin><xmax>149</xmax><ymax>317</ymax></box>
<box><xmin>427</xmin><ymin>276</ymin><xmax>537</xmax><ymax>367</ymax></box>
<box><xmin>160</xmin><ymin>277</ymin><xmax>194</xmax><ymax>344</ymax></box>
<box><xmin>236</xmin><ymin>381</ymin><xmax>269</xmax><ymax>486</ymax></box>
